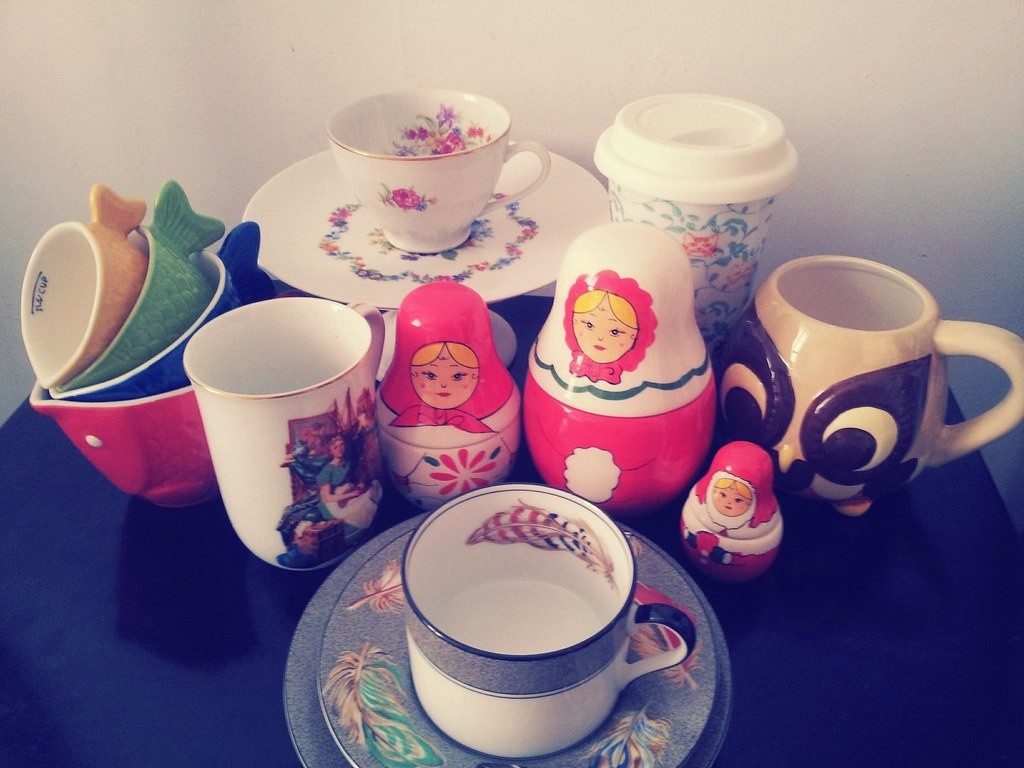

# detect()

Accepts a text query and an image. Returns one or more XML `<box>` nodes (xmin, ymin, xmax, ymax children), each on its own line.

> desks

<box><xmin>0</xmin><ymin>297</ymin><xmax>1024</xmax><ymax>768</ymax></box>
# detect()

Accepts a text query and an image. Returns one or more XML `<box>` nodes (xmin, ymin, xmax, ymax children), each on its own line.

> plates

<box><xmin>244</xmin><ymin>144</ymin><xmax>609</xmax><ymax>310</ymax></box>
<box><xmin>287</xmin><ymin>509</ymin><xmax>731</xmax><ymax>768</ymax></box>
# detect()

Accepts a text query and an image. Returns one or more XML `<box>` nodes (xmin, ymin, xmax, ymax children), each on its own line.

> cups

<box><xmin>21</xmin><ymin>184</ymin><xmax>147</xmax><ymax>389</ymax></box>
<box><xmin>722</xmin><ymin>255</ymin><xmax>1024</xmax><ymax>516</ymax></box>
<box><xmin>403</xmin><ymin>483</ymin><xmax>698</xmax><ymax>754</ymax></box>
<box><xmin>50</xmin><ymin>181</ymin><xmax>225</xmax><ymax>385</ymax></box>
<box><xmin>53</xmin><ymin>223</ymin><xmax>277</xmax><ymax>401</ymax></box>
<box><xmin>595</xmin><ymin>95</ymin><xmax>798</xmax><ymax>354</ymax></box>
<box><xmin>30</xmin><ymin>378</ymin><xmax>222</xmax><ymax>507</ymax></box>
<box><xmin>181</xmin><ymin>297</ymin><xmax>385</xmax><ymax>573</ymax></box>
<box><xmin>324</xmin><ymin>91</ymin><xmax>512</xmax><ymax>254</ymax></box>
